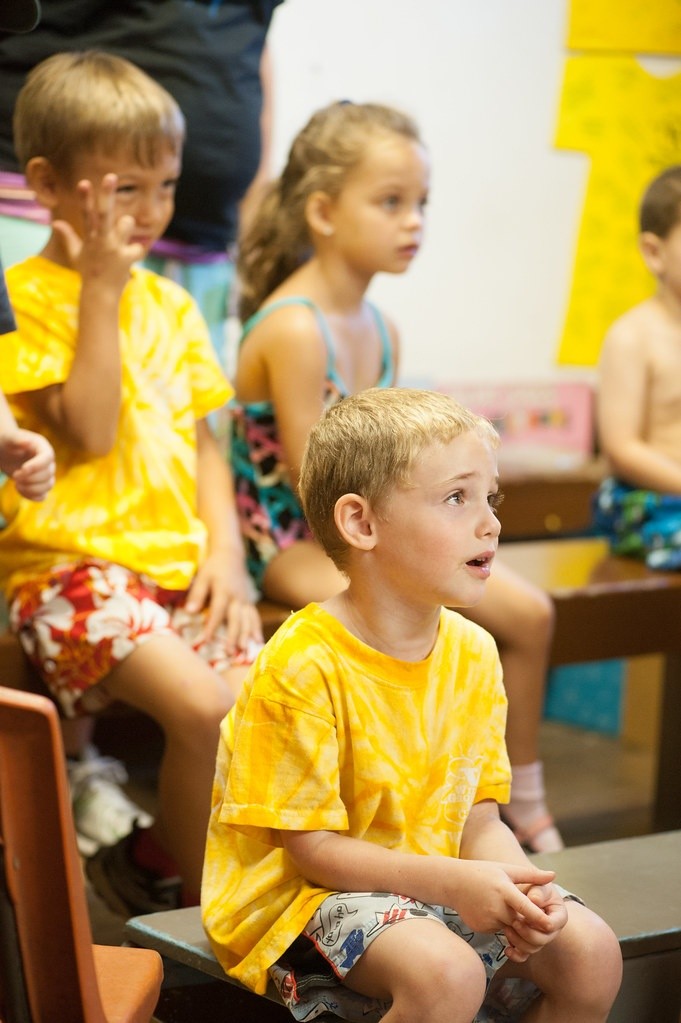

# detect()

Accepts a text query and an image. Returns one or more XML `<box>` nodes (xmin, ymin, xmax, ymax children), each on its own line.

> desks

<box><xmin>100</xmin><ymin>537</ymin><xmax>681</xmax><ymax>833</ymax></box>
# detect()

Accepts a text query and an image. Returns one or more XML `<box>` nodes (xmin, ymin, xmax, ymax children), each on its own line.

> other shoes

<box><xmin>84</xmin><ymin>818</ymin><xmax>221</xmax><ymax>985</ymax></box>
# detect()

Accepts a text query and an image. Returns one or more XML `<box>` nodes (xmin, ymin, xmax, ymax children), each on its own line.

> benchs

<box><xmin>125</xmin><ymin>830</ymin><xmax>681</xmax><ymax>1009</ymax></box>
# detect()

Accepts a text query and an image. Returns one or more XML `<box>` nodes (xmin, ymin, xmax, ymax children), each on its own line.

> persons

<box><xmin>0</xmin><ymin>0</ymin><xmax>287</xmax><ymax>367</ymax></box>
<box><xmin>0</xmin><ymin>51</ymin><xmax>267</xmax><ymax>914</ymax></box>
<box><xmin>0</xmin><ymin>261</ymin><xmax>56</xmax><ymax>501</ymax></box>
<box><xmin>231</xmin><ymin>100</ymin><xmax>562</xmax><ymax>857</ymax></box>
<box><xmin>594</xmin><ymin>168</ymin><xmax>680</xmax><ymax>572</ymax></box>
<box><xmin>198</xmin><ymin>388</ymin><xmax>622</xmax><ymax>1023</ymax></box>
